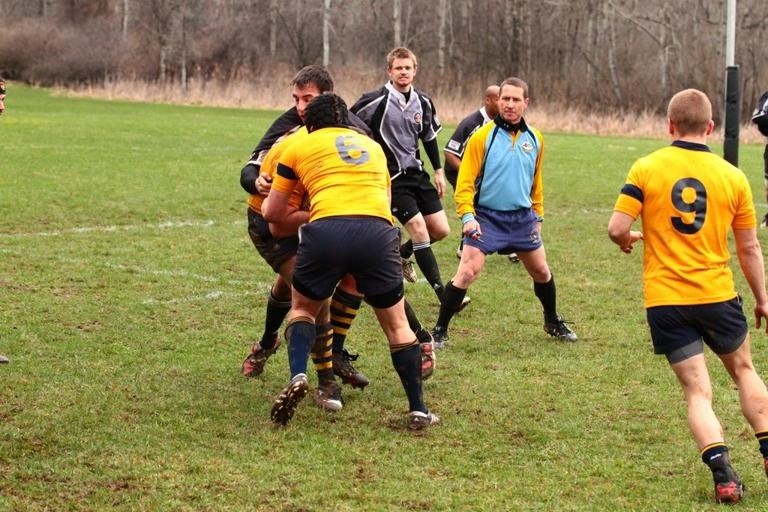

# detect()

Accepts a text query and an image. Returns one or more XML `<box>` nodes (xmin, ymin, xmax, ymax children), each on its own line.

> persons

<box><xmin>262</xmin><ymin>91</ymin><xmax>442</xmax><ymax>432</ymax></box>
<box><xmin>605</xmin><ymin>86</ymin><xmax>768</xmax><ymax>504</ymax></box>
<box><xmin>428</xmin><ymin>76</ymin><xmax>581</xmax><ymax>347</ymax></box>
<box><xmin>750</xmin><ymin>90</ymin><xmax>768</xmax><ymax>230</ymax></box>
<box><xmin>442</xmin><ymin>84</ymin><xmax>522</xmax><ymax>266</ymax></box>
<box><xmin>240</xmin><ymin>63</ymin><xmax>437</xmax><ymax>384</ymax></box>
<box><xmin>347</xmin><ymin>44</ymin><xmax>473</xmax><ymax>315</ymax></box>
<box><xmin>246</xmin><ymin>117</ymin><xmax>370</xmax><ymax>412</ymax></box>
<box><xmin>0</xmin><ymin>76</ymin><xmax>10</xmax><ymax>365</ymax></box>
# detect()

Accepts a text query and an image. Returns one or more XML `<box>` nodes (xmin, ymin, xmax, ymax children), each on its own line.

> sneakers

<box><xmin>241</xmin><ymin>331</ymin><xmax>281</xmax><ymax>377</ymax></box>
<box><xmin>760</xmin><ymin>445</ymin><xmax>768</xmax><ymax>480</ymax></box>
<box><xmin>508</xmin><ymin>252</ymin><xmax>519</xmax><ymax>263</ymax></box>
<box><xmin>313</xmin><ymin>380</ymin><xmax>345</xmax><ymax>410</ymax></box>
<box><xmin>457</xmin><ymin>239</ymin><xmax>465</xmax><ymax>258</ymax></box>
<box><xmin>455</xmin><ymin>296</ymin><xmax>470</xmax><ymax>312</ymax></box>
<box><xmin>270</xmin><ymin>372</ymin><xmax>310</xmax><ymax>425</ymax></box>
<box><xmin>708</xmin><ymin>451</ymin><xmax>745</xmax><ymax>505</ymax></box>
<box><xmin>409</xmin><ymin>409</ymin><xmax>441</xmax><ymax>429</ymax></box>
<box><xmin>332</xmin><ymin>347</ymin><xmax>370</xmax><ymax>388</ymax></box>
<box><xmin>431</xmin><ymin>325</ymin><xmax>447</xmax><ymax>349</ymax></box>
<box><xmin>543</xmin><ymin>315</ymin><xmax>578</xmax><ymax>342</ymax></box>
<box><xmin>419</xmin><ymin>329</ymin><xmax>435</xmax><ymax>380</ymax></box>
<box><xmin>400</xmin><ymin>245</ymin><xmax>417</xmax><ymax>283</ymax></box>
